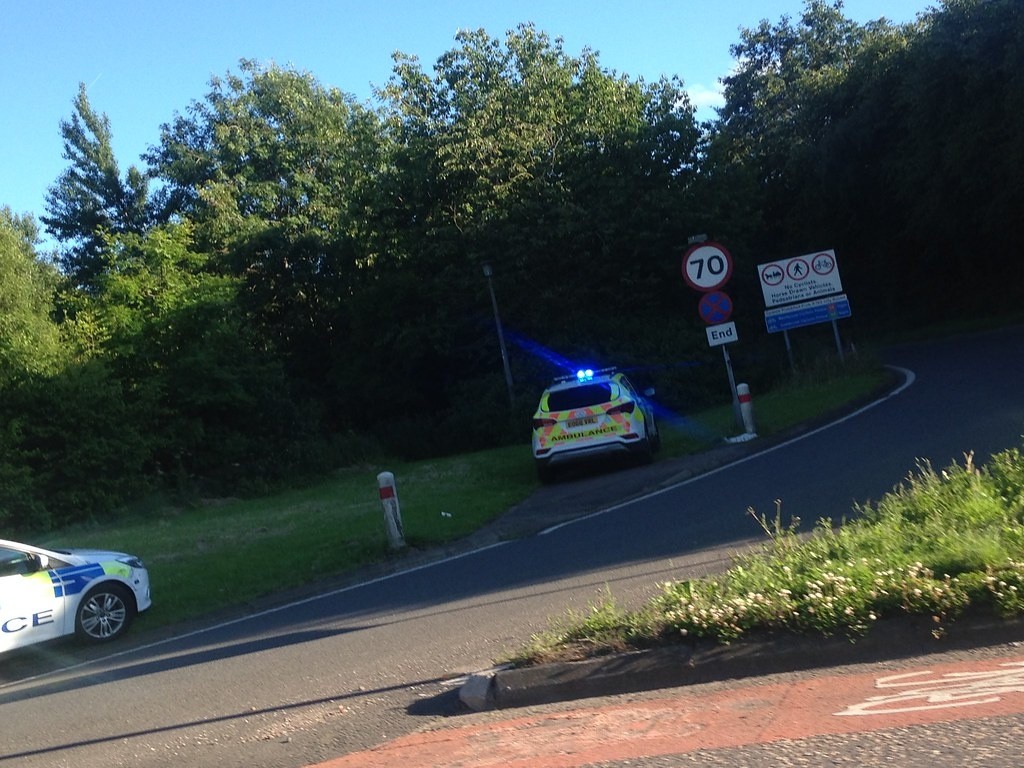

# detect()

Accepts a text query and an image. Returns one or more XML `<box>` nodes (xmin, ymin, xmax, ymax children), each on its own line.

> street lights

<box><xmin>480</xmin><ymin>257</ymin><xmax>521</xmax><ymax>446</ymax></box>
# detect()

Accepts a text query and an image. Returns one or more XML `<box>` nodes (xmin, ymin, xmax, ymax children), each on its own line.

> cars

<box><xmin>530</xmin><ymin>365</ymin><xmax>661</xmax><ymax>481</ymax></box>
<box><xmin>0</xmin><ymin>539</ymin><xmax>152</xmax><ymax>654</ymax></box>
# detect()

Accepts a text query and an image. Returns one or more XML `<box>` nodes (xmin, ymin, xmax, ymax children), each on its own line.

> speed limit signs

<box><xmin>681</xmin><ymin>243</ymin><xmax>733</xmax><ymax>292</ymax></box>
